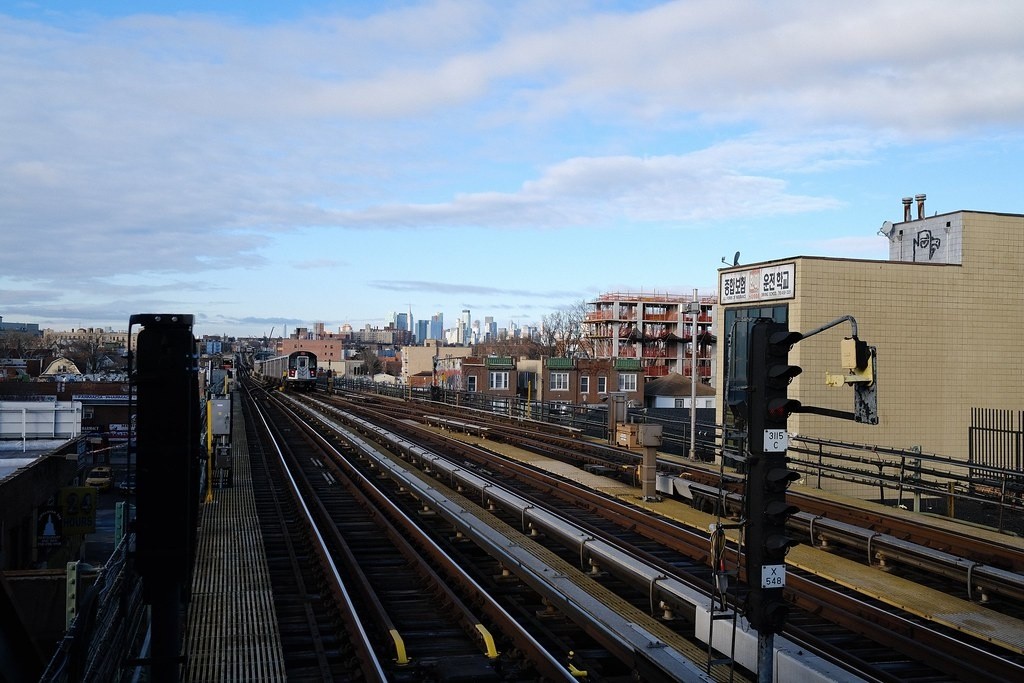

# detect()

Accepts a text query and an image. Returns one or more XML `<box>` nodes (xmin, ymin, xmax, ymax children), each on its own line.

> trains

<box><xmin>253</xmin><ymin>350</ymin><xmax>317</xmax><ymax>391</ymax></box>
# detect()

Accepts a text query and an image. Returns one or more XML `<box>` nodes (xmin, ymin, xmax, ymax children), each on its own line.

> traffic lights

<box><xmin>743</xmin><ymin>458</ymin><xmax>803</xmax><ymax>631</ymax></box>
<box><xmin>746</xmin><ymin>316</ymin><xmax>804</xmax><ymax>460</ymax></box>
